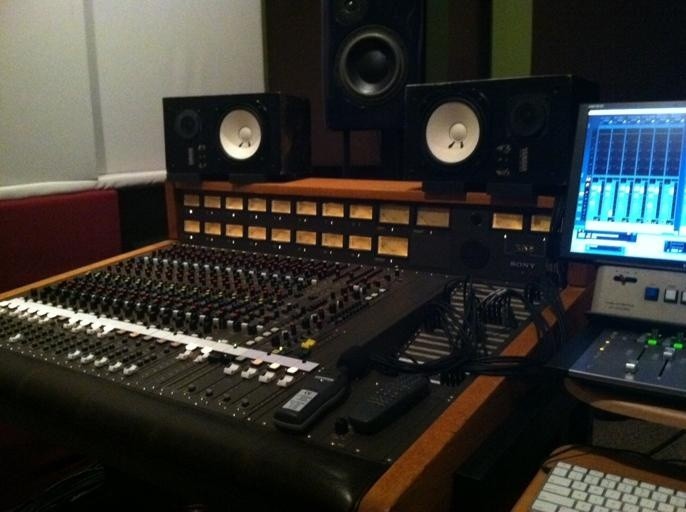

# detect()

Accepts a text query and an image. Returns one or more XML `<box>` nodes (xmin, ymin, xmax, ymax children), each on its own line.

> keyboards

<box><xmin>529</xmin><ymin>458</ymin><xmax>686</xmax><ymax>512</ymax></box>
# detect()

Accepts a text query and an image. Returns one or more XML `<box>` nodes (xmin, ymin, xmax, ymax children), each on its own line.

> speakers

<box><xmin>400</xmin><ymin>74</ymin><xmax>578</xmax><ymax>205</ymax></box>
<box><xmin>160</xmin><ymin>90</ymin><xmax>312</xmax><ymax>186</ymax></box>
<box><xmin>321</xmin><ymin>1</ymin><xmax>424</xmax><ymax>132</ymax></box>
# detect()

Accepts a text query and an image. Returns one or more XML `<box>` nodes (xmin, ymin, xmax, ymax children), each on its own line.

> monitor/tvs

<box><xmin>553</xmin><ymin>97</ymin><xmax>686</xmax><ymax>272</ymax></box>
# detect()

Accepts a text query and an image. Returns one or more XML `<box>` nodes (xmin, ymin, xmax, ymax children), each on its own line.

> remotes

<box><xmin>344</xmin><ymin>373</ymin><xmax>430</xmax><ymax>436</ymax></box>
<box><xmin>275</xmin><ymin>366</ymin><xmax>352</xmax><ymax>436</ymax></box>
